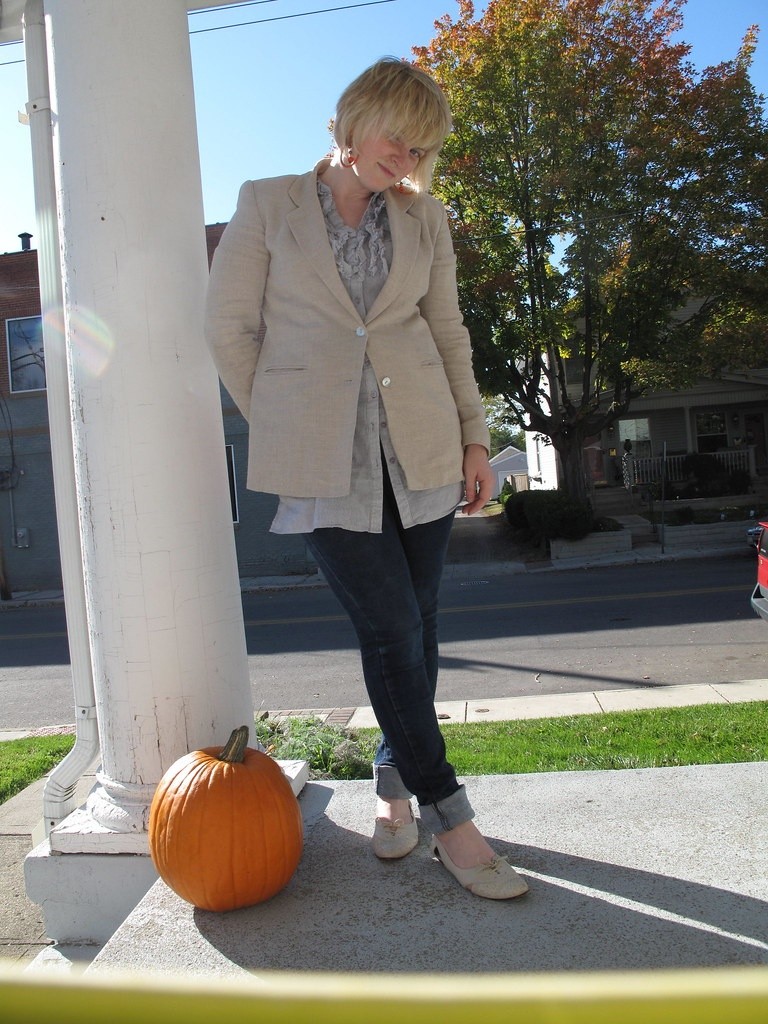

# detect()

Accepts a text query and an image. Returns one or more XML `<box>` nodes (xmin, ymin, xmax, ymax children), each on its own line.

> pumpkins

<box><xmin>148</xmin><ymin>725</ymin><xmax>303</xmax><ymax>912</ymax></box>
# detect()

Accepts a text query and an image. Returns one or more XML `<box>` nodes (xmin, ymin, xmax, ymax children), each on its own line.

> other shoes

<box><xmin>372</xmin><ymin>798</ymin><xmax>419</xmax><ymax>858</ymax></box>
<box><xmin>431</xmin><ymin>834</ymin><xmax>528</xmax><ymax>899</ymax></box>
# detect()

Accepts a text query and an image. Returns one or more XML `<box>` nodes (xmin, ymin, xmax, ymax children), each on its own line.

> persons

<box><xmin>204</xmin><ymin>61</ymin><xmax>530</xmax><ymax>900</ymax></box>
